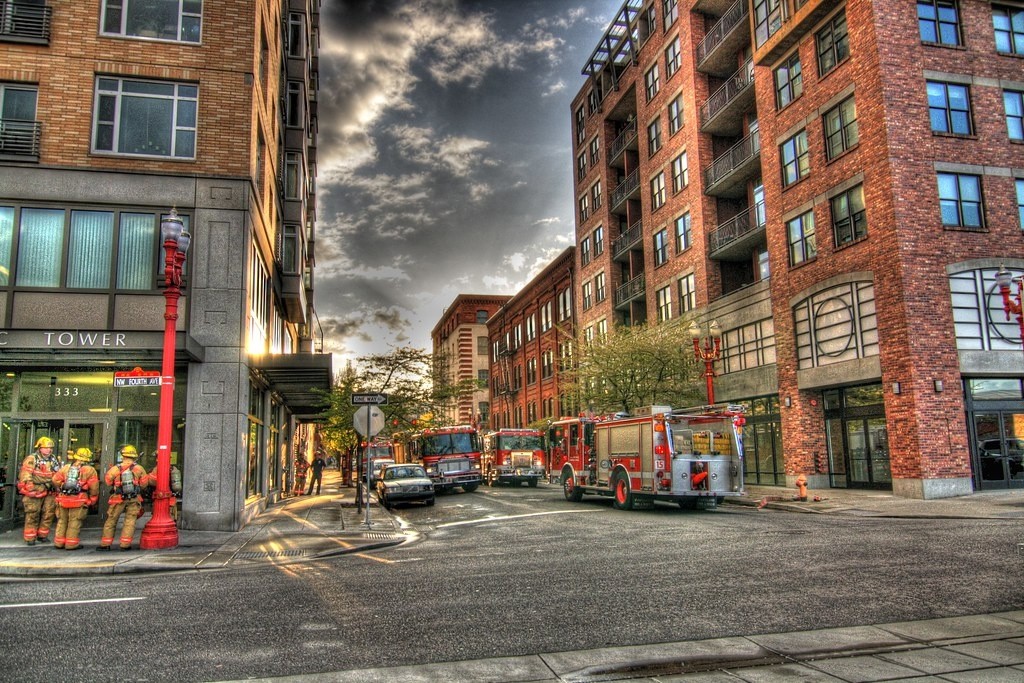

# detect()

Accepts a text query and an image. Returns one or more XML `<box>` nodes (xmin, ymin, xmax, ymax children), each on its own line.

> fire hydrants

<box><xmin>794</xmin><ymin>473</ymin><xmax>809</xmax><ymax>502</ymax></box>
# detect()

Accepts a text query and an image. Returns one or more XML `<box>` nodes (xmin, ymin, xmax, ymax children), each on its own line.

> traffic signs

<box><xmin>350</xmin><ymin>393</ymin><xmax>389</xmax><ymax>407</ymax></box>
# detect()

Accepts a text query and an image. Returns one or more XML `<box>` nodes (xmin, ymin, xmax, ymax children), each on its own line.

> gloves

<box><xmin>90</xmin><ymin>495</ymin><xmax>98</xmax><ymax>505</ymax></box>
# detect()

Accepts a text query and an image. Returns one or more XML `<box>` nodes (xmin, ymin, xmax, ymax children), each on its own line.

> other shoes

<box><xmin>121</xmin><ymin>546</ymin><xmax>132</xmax><ymax>551</ymax></box>
<box><xmin>64</xmin><ymin>545</ymin><xmax>83</xmax><ymax>550</ymax></box>
<box><xmin>96</xmin><ymin>546</ymin><xmax>110</xmax><ymax>551</ymax></box>
<box><xmin>36</xmin><ymin>536</ymin><xmax>50</xmax><ymax>543</ymax></box>
<box><xmin>25</xmin><ymin>540</ymin><xmax>35</xmax><ymax>545</ymax></box>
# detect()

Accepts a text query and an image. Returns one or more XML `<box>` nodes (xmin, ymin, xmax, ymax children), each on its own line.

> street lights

<box><xmin>688</xmin><ymin>319</ymin><xmax>722</xmax><ymax>406</ymax></box>
<box><xmin>137</xmin><ymin>204</ymin><xmax>190</xmax><ymax>549</ymax></box>
<box><xmin>993</xmin><ymin>262</ymin><xmax>1024</xmax><ymax>351</ymax></box>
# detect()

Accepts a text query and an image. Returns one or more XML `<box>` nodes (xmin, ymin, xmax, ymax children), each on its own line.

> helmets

<box><xmin>34</xmin><ymin>437</ymin><xmax>55</xmax><ymax>448</ymax></box>
<box><xmin>299</xmin><ymin>450</ymin><xmax>307</xmax><ymax>457</ymax></box>
<box><xmin>148</xmin><ymin>450</ymin><xmax>172</xmax><ymax>460</ymax></box>
<box><xmin>72</xmin><ymin>447</ymin><xmax>92</xmax><ymax>463</ymax></box>
<box><xmin>122</xmin><ymin>445</ymin><xmax>138</xmax><ymax>458</ymax></box>
<box><xmin>315</xmin><ymin>451</ymin><xmax>322</xmax><ymax>456</ymax></box>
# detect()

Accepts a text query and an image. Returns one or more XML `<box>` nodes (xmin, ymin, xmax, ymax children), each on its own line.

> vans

<box><xmin>369</xmin><ymin>459</ymin><xmax>396</xmax><ymax>490</ymax></box>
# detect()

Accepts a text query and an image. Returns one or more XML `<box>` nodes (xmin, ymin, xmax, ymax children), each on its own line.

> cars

<box><xmin>376</xmin><ymin>463</ymin><xmax>437</xmax><ymax>511</ymax></box>
<box><xmin>979</xmin><ymin>438</ymin><xmax>1024</xmax><ymax>480</ymax></box>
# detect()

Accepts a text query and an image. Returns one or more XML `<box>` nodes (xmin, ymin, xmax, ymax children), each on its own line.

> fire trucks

<box><xmin>393</xmin><ymin>426</ymin><xmax>485</xmax><ymax>496</ymax></box>
<box><xmin>481</xmin><ymin>427</ymin><xmax>546</xmax><ymax>488</ymax></box>
<box><xmin>549</xmin><ymin>404</ymin><xmax>747</xmax><ymax>513</ymax></box>
<box><xmin>360</xmin><ymin>437</ymin><xmax>394</xmax><ymax>483</ymax></box>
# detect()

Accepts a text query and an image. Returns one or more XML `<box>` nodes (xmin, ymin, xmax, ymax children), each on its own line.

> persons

<box><xmin>52</xmin><ymin>447</ymin><xmax>99</xmax><ymax>551</ymax></box>
<box><xmin>294</xmin><ymin>452</ymin><xmax>309</xmax><ymax>496</ymax></box>
<box><xmin>17</xmin><ymin>437</ymin><xmax>62</xmax><ymax>546</ymax></box>
<box><xmin>148</xmin><ymin>450</ymin><xmax>176</xmax><ymax>523</ymax></box>
<box><xmin>306</xmin><ymin>451</ymin><xmax>326</xmax><ymax>495</ymax></box>
<box><xmin>96</xmin><ymin>444</ymin><xmax>147</xmax><ymax>552</ymax></box>
